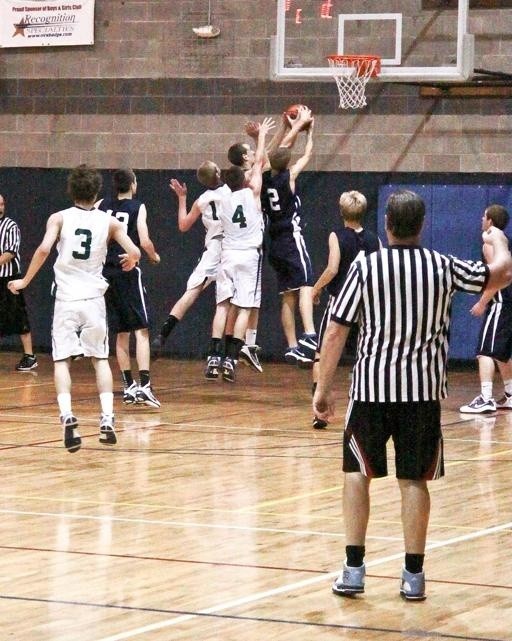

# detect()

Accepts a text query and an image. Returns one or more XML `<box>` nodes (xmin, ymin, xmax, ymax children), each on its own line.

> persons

<box><xmin>311</xmin><ymin>191</ymin><xmax>384</xmax><ymax>430</ymax></box>
<box><xmin>312</xmin><ymin>188</ymin><xmax>512</xmax><ymax>601</ymax></box>
<box><xmin>203</xmin><ymin>116</ymin><xmax>276</xmax><ymax>384</ymax></box>
<box><xmin>150</xmin><ymin>121</ymin><xmax>265</xmax><ymax>372</ymax></box>
<box><xmin>227</xmin><ymin>104</ymin><xmax>313</xmax><ymax>375</ymax></box>
<box><xmin>460</xmin><ymin>203</ymin><xmax>511</xmax><ymax>414</ymax></box>
<box><xmin>261</xmin><ymin>107</ymin><xmax>323</xmax><ymax>370</ymax></box>
<box><xmin>7</xmin><ymin>163</ymin><xmax>141</xmax><ymax>454</ymax></box>
<box><xmin>0</xmin><ymin>195</ymin><xmax>38</xmax><ymax>372</ymax></box>
<box><xmin>94</xmin><ymin>164</ymin><xmax>162</xmax><ymax>409</ymax></box>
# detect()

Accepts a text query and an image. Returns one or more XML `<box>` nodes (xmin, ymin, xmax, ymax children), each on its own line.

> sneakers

<box><xmin>398</xmin><ymin>566</ymin><xmax>428</xmax><ymax>602</ymax></box>
<box><xmin>495</xmin><ymin>392</ymin><xmax>512</xmax><ymax>410</ymax></box>
<box><xmin>220</xmin><ymin>356</ymin><xmax>236</xmax><ymax>385</ymax></box>
<box><xmin>242</xmin><ymin>342</ymin><xmax>263</xmax><ymax>374</ymax></box>
<box><xmin>283</xmin><ymin>346</ymin><xmax>315</xmax><ymax>368</ymax></box>
<box><xmin>122</xmin><ymin>379</ymin><xmax>138</xmax><ymax>406</ymax></box>
<box><xmin>331</xmin><ymin>561</ymin><xmax>367</xmax><ymax>597</ymax></box>
<box><xmin>98</xmin><ymin>413</ymin><xmax>119</xmax><ymax>446</ymax></box>
<box><xmin>134</xmin><ymin>380</ymin><xmax>162</xmax><ymax>410</ymax></box>
<box><xmin>150</xmin><ymin>334</ymin><xmax>166</xmax><ymax>363</ymax></box>
<box><xmin>15</xmin><ymin>353</ymin><xmax>40</xmax><ymax>372</ymax></box>
<box><xmin>59</xmin><ymin>411</ymin><xmax>83</xmax><ymax>455</ymax></box>
<box><xmin>297</xmin><ymin>331</ymin><xmax>319</xmax><ymax>351</ymax></box>
<box><xmin>459</xmin><ymin>394</ymin><xmax>497</xmax><ymax>416</ymax></box>
<box><xmin>204</xmin><ymin>353</ymin><xmax>221</xmax><ymax>380</ymax></box>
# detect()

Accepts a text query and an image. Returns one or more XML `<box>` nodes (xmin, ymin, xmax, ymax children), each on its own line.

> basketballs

<box><xmin>286</xmin><ymin>104</ymin><xmax>311</xmax><ymax>131</ymax></box>
